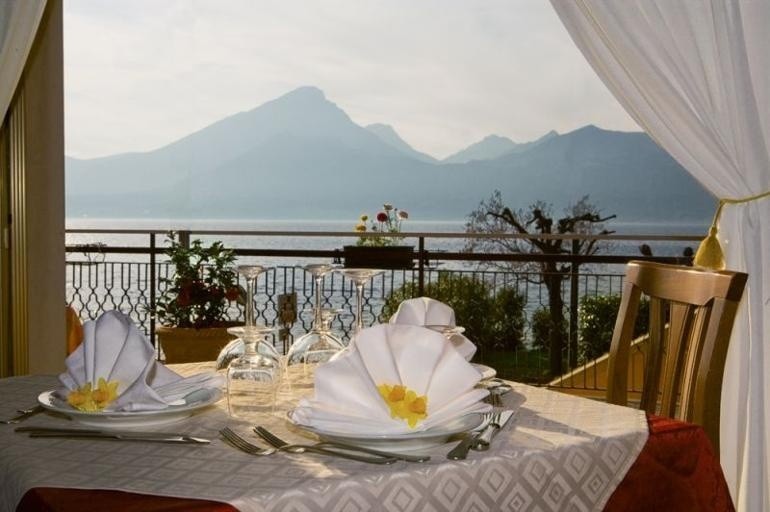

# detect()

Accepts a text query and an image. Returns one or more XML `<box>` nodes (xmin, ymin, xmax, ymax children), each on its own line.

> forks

<box><xmin>219</xmin><ymin>427</ymin><xmax>398</xmax><ymax>466</ymax></box>
<box><xmin>253</xmin><ymin>426</ymin><xmax>432</xmax><ymax>462</ymax></box>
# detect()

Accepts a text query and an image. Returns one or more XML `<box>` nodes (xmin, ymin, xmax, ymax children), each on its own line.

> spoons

<box><xmin>482</xmin><ymin>378</ymin><xmax>511</xmax><ymax>411</ymax></box>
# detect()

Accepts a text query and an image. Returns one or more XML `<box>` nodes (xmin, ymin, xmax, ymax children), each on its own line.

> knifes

<box><xmin>30</xmin><ymin>427</ymin><xmax>212</xmax><ymax>447</ymax></box>
<box><xmin>471</xmin><ymin>408</ymin><xmax>516</xmax><ymax>451</ymax></box>
<box><xmin>446</xmin><ymin>412</ymin><xmax>495</xmax><ymax>462</ymax></box>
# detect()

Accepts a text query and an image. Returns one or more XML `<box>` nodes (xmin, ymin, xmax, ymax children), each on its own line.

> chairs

<box><xmin>608</xmin><ymin>259</ymin><xmax>749</xmax><ymax>462</ymax></box>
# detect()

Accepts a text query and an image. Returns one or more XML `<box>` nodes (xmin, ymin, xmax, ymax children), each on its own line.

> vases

<box><xmin>153</xmin><ymin>322</ymin><xmax>247</xmax><ymax>365</ymax></box>
<box><xmin>343</xmin><ymin>244</ymin><xmax>415</xmax><ymax>268</ymax></box>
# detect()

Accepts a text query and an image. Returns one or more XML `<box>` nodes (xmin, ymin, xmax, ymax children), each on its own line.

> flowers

<box><xmin>140</xmin><ymin>228</ymin><xmax>247</xmax><ymax>329</ymax></box>
<box><xmin>354</xmin><ymin>202</ymin><xmax>408</xmax><ymax>247</ymax></box>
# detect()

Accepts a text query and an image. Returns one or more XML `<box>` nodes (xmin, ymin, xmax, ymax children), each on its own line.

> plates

<box><xmin>287</xmin><ymin>408</ymin><xmax>483</xmax><ymax>449</ymax></box>
<box><xmin>38</xmin><ymin>387</ymin><xmax>225</xmax><ymax>427</ymax></box>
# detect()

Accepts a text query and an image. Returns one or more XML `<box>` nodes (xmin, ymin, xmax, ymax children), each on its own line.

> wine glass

<box><xmin>216</xmin><ymin>264</ymin><xmax>282</xmax><ymax>397</ymax></box>
<box><xmin>328</xmin><ymin>267</ymin><xmax>388</xmax><ymax>339</ymax></box>
<box><xmin>285</xmin><ymin>263</ymin><xmax>345</xmax><ymax>400</ymax></box>
<box><xmin>227</xmin><ymin>323</ymin><xmax>278</xmax><ymax>421</ymax></box>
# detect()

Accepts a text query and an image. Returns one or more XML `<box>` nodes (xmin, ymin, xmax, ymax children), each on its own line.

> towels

<box><xmin>289</xmin><ymin>296</ymin><xmax>498</xmax><ymax>437</ymax></box>
<box><xmin>48</xmin><ymin>310</ymin><xmax>229</xmax><ymax>412</ymax></box>
